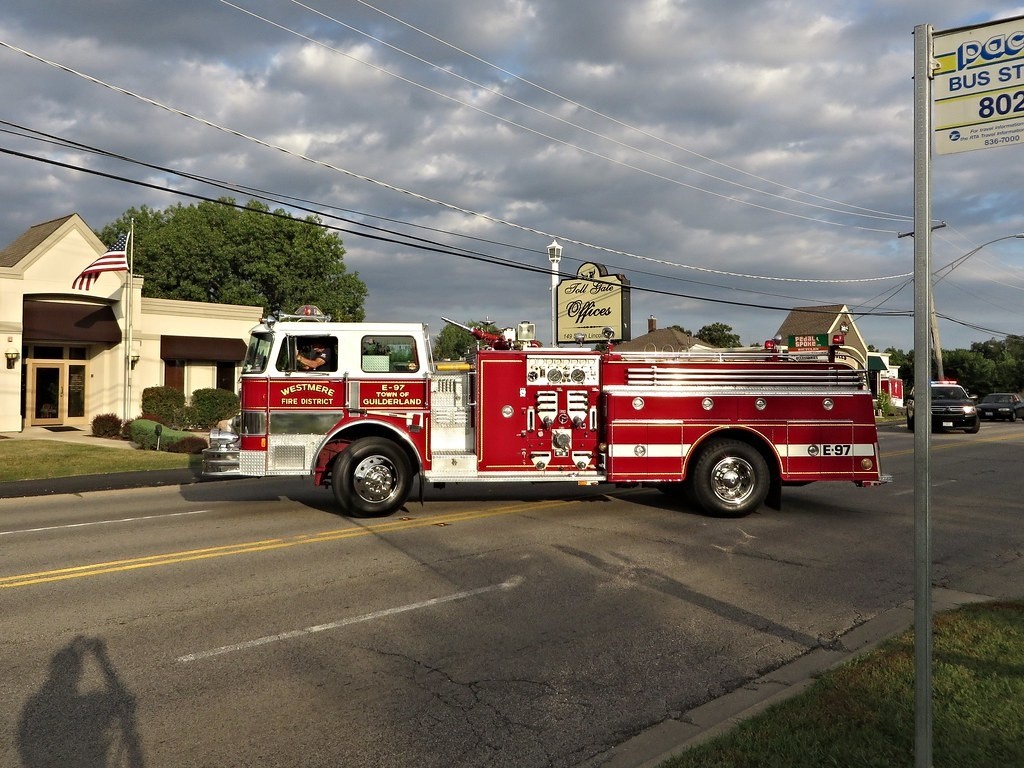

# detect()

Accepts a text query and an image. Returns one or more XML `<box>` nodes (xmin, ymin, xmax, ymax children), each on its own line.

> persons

<box><xmin>296</xmin><ymin>339</ymin><xmax>338</xmax><ymax>372</ymax></box>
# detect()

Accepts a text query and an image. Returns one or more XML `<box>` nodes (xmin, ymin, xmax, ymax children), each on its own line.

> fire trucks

<box><xmin>201</xmin><ymin>305</ymin><xmax>895</xmax><ymax>519</ymax></box>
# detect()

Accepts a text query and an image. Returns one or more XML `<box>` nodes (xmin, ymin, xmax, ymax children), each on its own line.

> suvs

<box><xmin>906</xmin><ymin>383</ymin><xmax>981</xmax><ymax>434</ymax></box>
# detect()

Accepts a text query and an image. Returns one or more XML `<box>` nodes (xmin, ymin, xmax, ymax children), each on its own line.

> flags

<box><xmin>72</xmin><ymin>229</ymin><xmax>131</xmax><ymax>290</ymax></box>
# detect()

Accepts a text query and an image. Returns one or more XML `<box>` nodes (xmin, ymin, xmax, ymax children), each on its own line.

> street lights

<box><xmin>927</xmin><ymin>232</ymin><xmax>1024</xmax><ymax>384</ymax></box>
<box><xmin>547</xmin><ymin>240</ymin><xmax>563</xmax><ymax>348</ymax></box>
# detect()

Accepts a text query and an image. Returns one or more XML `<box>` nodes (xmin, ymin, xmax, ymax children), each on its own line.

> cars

<box><xmin>975</xmin><ymin>393</ymin><xmax>1024</xmax><ymax>421</ymax></box>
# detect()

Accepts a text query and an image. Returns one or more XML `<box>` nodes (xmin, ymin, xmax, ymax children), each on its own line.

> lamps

<box><xmin>4</xmin><ymin>347</ymin><xmax>20</xmax><ymax>369</ymax></box>
<box><xmin>127</xmin><ymin>351</ymin><xmax>140</xmax><ymax>370</ymax></box>
